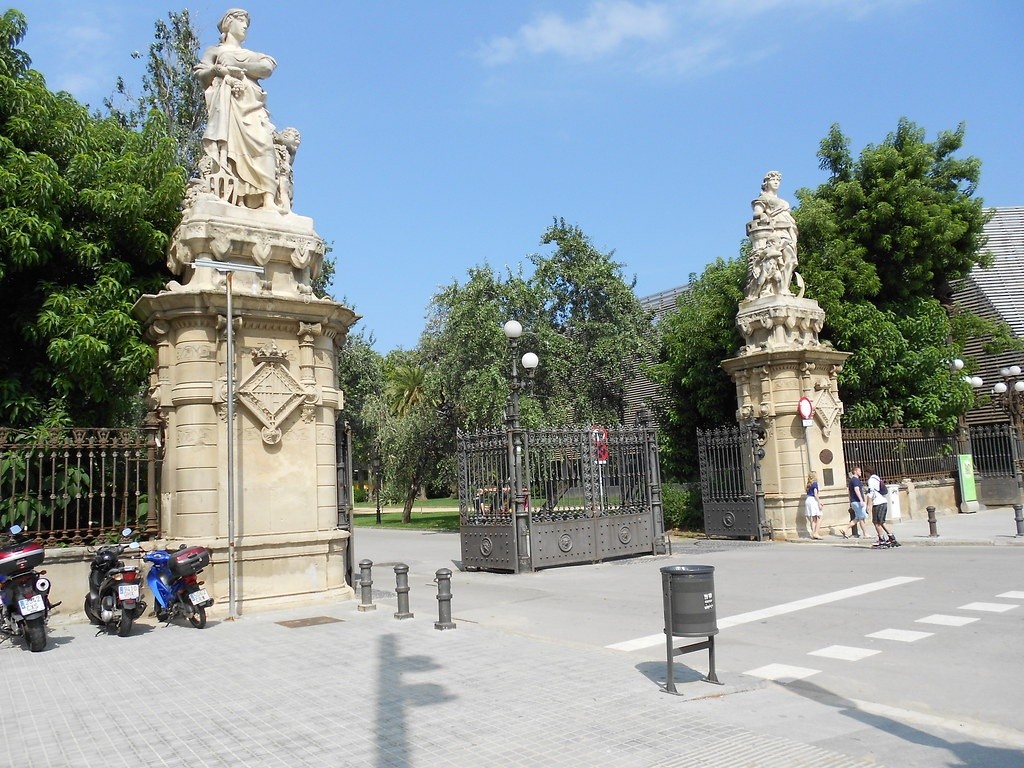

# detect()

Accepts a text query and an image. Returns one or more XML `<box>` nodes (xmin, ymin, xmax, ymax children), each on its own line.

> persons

<box><xmin>749</xmin><ymin>169</ymin><xmax>806</xmax><ymax>301</ymax></box>
<box><xmin>802</xmin><ymin>471</ymin><xmax>824</xmax><ymax>540</ymax></box>
<box><xmin>839</xmin><ymin>467</ymin><xmax>874</xmax><ymax>539</ymax></box>
<box><xmin>862</xmin><ymin>464</ymin><xmax>901</xmax><ymax>548</ymax></box>
<box><xmin>185</xmin><ymin>7</ymin><xmax>300</xmax><ymax>215</ymax></box>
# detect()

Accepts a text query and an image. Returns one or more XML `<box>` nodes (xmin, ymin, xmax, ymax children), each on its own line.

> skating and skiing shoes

<box><xmin>872</xmin><ymin>535</ymin><xmax>890</xmax><ymax>549</ymax></box>
<box><xmin>885</xmin><ymin>533</ymin><xmax>901</xmax><ymax>547</ymax></box>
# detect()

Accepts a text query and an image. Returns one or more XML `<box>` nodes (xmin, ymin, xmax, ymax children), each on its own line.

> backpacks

<box><xmin>870</xmin><ymin>477</ymin><xmax>888</xmax><ymax>496</ymax></box>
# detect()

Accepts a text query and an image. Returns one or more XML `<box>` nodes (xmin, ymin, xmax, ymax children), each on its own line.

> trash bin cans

<box><xmin>658</xmin><ymin>564</ymin><xmax>724</xmax><ymax>696</ymax></box>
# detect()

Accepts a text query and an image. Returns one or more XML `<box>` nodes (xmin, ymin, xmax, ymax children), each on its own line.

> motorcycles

<box><xmin>129</xmin><ymin>541</ymin><xmax>214</xmax><ymax>629</ymax></box>
<box><xmin>0</xmin><ymin>525</ymin><xmax>63</xmax><ymax>653</ymax></box>
<box><xmin>85</xmin><ymin>526</ymin><xmax>147</xmax><ymax>636</ymax></box>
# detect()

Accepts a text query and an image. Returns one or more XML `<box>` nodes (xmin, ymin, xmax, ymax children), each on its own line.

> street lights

<box><xmin>940</xmin><ymin>357</ymin><xmax>984</xmax><ymax>453</ymax></box>
<box><xmin>373</xmin><ymin>456</ymin><xmax>382</xmax><ymax>524</ymax></box>
<box><xmin>503</xmin><ymin>319</ymin><xmax>540</xmax><ymax>572</ymax></box>
<box><xmin>991</xmin><ymin>364</ymin><xmax>1024</xmax><ymax>503</ymax></box>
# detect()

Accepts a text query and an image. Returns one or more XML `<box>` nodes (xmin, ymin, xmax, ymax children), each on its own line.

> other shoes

<box><xmin>839</xmin><ymin>529</ymin><xmax>850</xmax><ymax>539</ymax></box>
<box><xmin>812</xmin><ymin>533</ymin><xmax>823</xmax><ymax>540</ymax></box>
<box><xmin>862</xmin><ymin>534</ymin><xmax>872</xmax><ymax>539</ymax></box>
